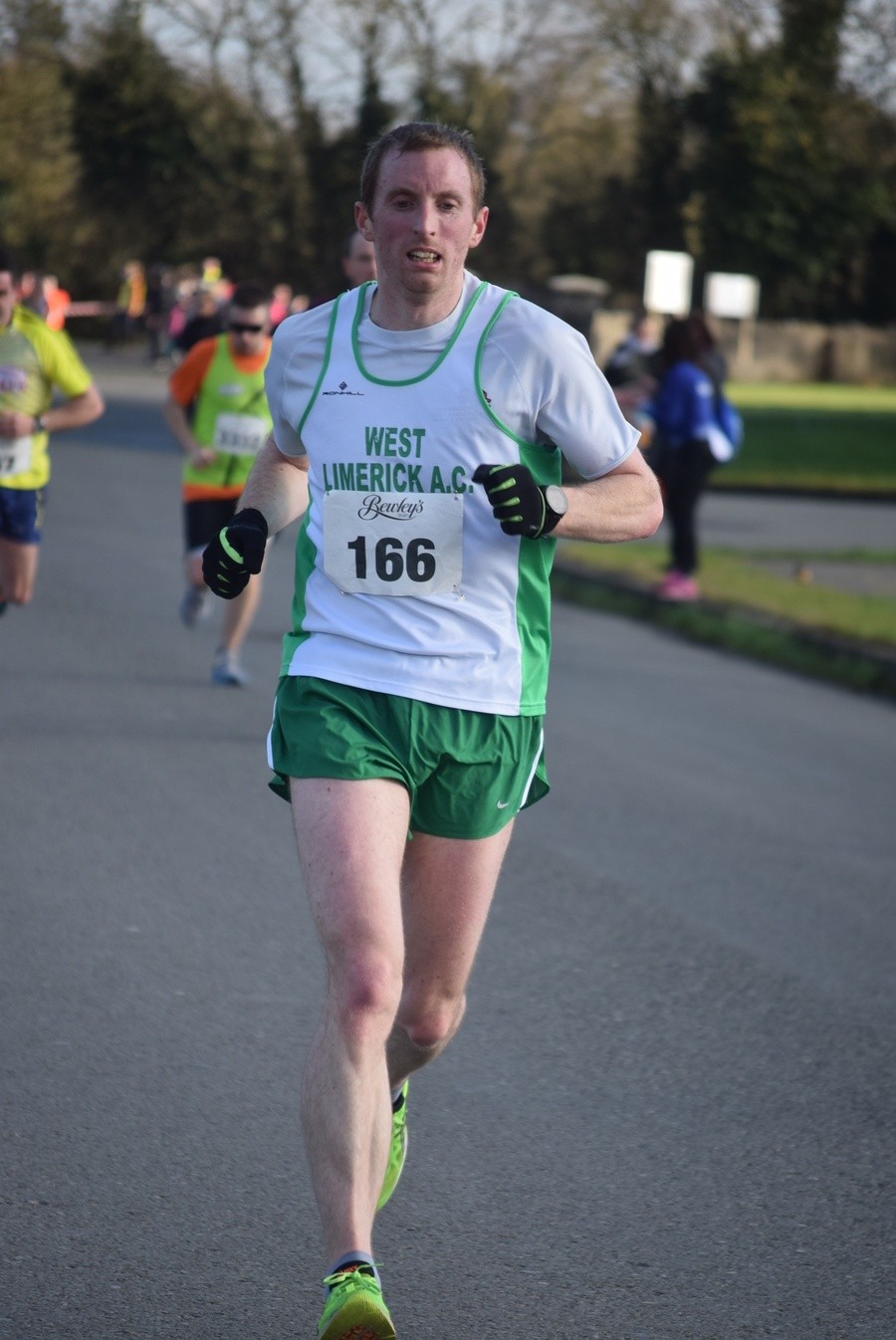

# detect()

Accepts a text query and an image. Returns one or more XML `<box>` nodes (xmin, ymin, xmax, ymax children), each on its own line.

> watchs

<box><xmin>538</xmin><ymin>483</ymin><xmax>571</xmax><ymax>537</ymax></box>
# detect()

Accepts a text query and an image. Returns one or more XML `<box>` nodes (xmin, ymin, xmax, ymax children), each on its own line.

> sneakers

<box><xmin>659</xmin><ymin>578</ymin><xmax>699</xmax><ymax>602</ymax></box>
<box><xmin>375</xmin><ymin>1079</ymin><xmax>409</xmax><ymax>1215</ymax></box>
<box><xmin>180</xmin><ymin>589</ymin><xmax>204</xmax><ymax>630</ymax></box>
<box><xmin>209</xmin><ymin>655</ymin><xmax>245</xmax><ymax>688</ymax></box>
<box><xmin>317</xmin><ymin>1259</ymin><xmax>397</xmax><ymax>1340</ymax></box>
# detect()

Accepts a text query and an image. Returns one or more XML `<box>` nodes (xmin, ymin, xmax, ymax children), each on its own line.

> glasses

<box><xmin>227</xmin><ymin>323</ymin><xmax>267</xmax><ymax>334</ymax></box>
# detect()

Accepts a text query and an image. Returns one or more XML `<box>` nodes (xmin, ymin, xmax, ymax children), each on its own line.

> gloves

<box><xmin>202</xmin><ymin>508</ymin><xmax>269</xmax><ymax>600</ymax></box>
<box><xmin>471</xmin><ymin>463</ymin><xmax>568</xmax><ymax>541</ymax></box>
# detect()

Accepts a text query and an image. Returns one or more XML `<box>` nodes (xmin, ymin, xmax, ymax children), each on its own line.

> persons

<box><xmin>2</xmin><ymin>249</ymin><xmax>725</xmax><ymax>689</ymax></box>
<box><xmin>199</xmin><ymin>118</ymin><xmax>672</xmax><ymax>1339</ymax></box>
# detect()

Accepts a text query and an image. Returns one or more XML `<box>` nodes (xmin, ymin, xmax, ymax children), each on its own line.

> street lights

<box><xmin>641</xmin><ymin>239</ymin><xmax>761</xmax><ymax>366</ymax></box>
